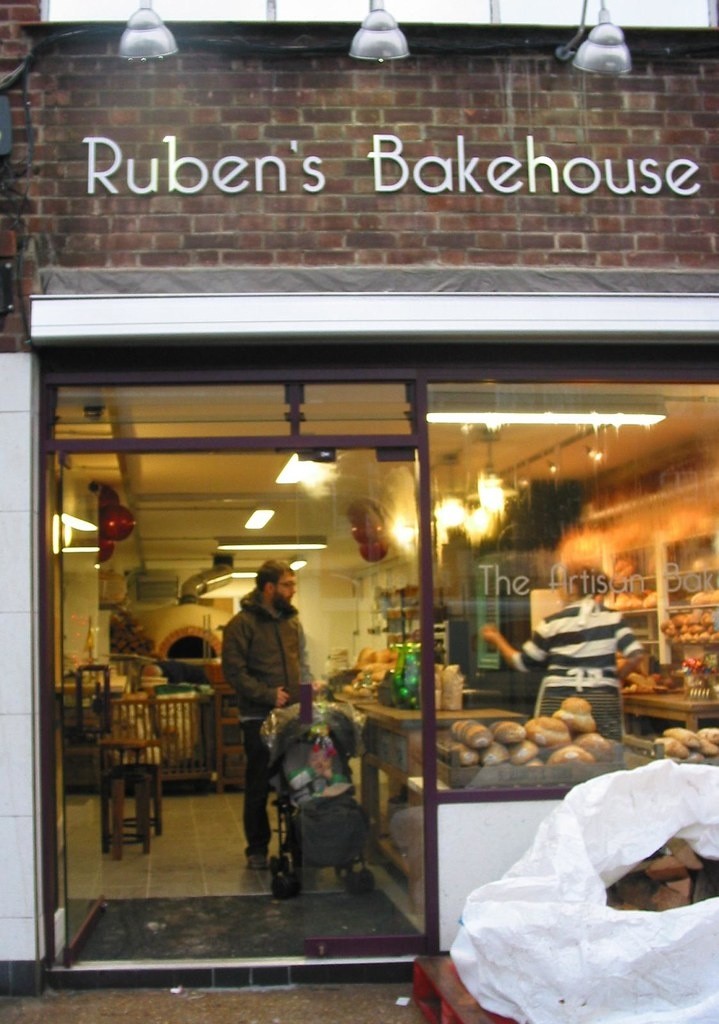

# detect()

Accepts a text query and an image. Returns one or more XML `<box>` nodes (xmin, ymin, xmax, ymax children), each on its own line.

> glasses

<box><xmin>277</xmin><ymin>582</ymin><xmax>295</xmax><ymax>586</ymax></box>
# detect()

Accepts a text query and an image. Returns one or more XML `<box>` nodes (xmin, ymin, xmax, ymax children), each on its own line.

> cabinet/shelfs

<box><xmin>601</xmin><ymin>530</ymin><xmax>719</xmax><ymax>667</ymax></box>
<box><xmin>216</xmin><ymin>684</ymin><xmax>246</xmax><ymax>794</ymax></box>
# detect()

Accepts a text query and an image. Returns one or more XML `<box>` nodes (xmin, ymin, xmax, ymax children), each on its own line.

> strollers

<box><xmin>259</xmin><ymin>684</ymin><xmax>378</xmax><ymax>904</ymax></box>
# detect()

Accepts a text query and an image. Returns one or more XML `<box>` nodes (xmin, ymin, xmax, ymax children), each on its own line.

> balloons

<box><xmin>346</xmin><ymin>496</ymin><xmax>396</xmax><ymax>560</ymax></box>
<box><xmin>96</xmin><ymin>484</ymin><xmax>136</xmax><ymax>564</ymax></box>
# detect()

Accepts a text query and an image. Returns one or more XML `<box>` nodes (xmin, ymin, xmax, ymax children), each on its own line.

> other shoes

<box><xmin>248</xmin><ymin>854</ymin><xmax>266</xmax><ymax>868</ymax></box>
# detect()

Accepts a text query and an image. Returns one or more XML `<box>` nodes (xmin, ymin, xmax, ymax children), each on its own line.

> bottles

<box><xmin>434</xmin><ymin>664</ymin><xmax>464</xmax><ymax>712</ymax></box>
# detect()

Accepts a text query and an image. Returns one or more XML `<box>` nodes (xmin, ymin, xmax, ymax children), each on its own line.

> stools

<box><xmin>98</xmin><ymin>699</ymin><xmax>162</xmax><ymax>859</ymax></box>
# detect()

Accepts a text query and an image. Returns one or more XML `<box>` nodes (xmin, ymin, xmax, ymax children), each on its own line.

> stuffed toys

<box><xmin>307</xmin><ymin>719</ymin><xmax>338</xmax><ymax>756</ymax></box>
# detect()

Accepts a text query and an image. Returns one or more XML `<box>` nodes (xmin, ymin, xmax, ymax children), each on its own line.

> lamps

<box><xmin>118</xmin><ymin>0</ymin><xmax>179</xmax><ymax>62</ymax></box>
<box><xmin>214</xmin><ymin>535</ymin><xmax>328</xmax><ymax>550</ymax></box>
<box><xmin>555</xmin><ymin>0</ymin><xmax>633</xmax><ymax>76</ymax></box>
<box><xmin>425</xmin><ymin>404</ymin><xmax>668</xmax><ymax>428</ymax></box>
<box><xmin>348</xmin><ymin>0</ymin><xmax>411</xmax><ymax>64</ymax></box>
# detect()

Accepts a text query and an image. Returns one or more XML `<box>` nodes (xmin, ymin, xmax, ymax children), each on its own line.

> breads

<box><xmin>655</xmin><ymin>727</ymin><xmax>719</xmax><ymax>762</ymax></box>
<box><xmin>660</xmin><ymin>613</ymin><xmax>719</xmax><ymax>642</ymax></box>
<box><xmin>613</xmin><ymin>555</ymin><xmax>657</xmax><ymax>609</ymax></box>
<box><xmin>436</xmin><ymin>698</ymin><xmax>611</xmax><ymax>766</ymax></box>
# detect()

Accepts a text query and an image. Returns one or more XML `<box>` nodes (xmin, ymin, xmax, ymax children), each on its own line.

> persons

<box><xmin>478</xmin><ymin>561</ymin><xmax>646</xmax><ymax>747</ymax></box>
<box><xmin>287</xmin><ymin>749</ymin><xmax>358</xmax><ymax>804</ymax></box>
<box><xmin>220</xmin><ymin>559</ymin><xmax>315</xmax><ymax>871</ymax></box>
